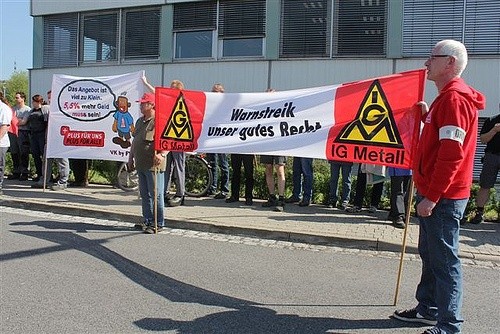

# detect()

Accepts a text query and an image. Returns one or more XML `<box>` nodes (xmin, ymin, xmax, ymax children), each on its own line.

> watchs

<box><xmin>161</xmin><ymin>151</ymin><xmax>167</xmax><ymax>158</ymax></box>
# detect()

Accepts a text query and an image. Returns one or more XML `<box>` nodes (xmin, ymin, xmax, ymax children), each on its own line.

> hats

<box><xmin>136</xmin><ymin>93</ymin><xmax>155</xmax><ymax>103</ymax></box>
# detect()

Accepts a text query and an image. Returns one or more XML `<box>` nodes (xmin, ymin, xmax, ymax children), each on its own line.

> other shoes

<box><xmin>226</xmin><ymin>193</ymin><xmax>239</xmax><ymax>203</ymax></box>
<box><xmin>167</xmin><ymin>196</ymin><xmax>184</xmax><ymax>206</ymax></box>
<box><xmin>299</xmin><ymin>199</ymin><xmax>309</xmax><ymax>207</ymax></box>
<box><xmin>0</xmin><ymin>173</ymin><xmax>89</xmax><ymax>191</ymax></box>
<box><xmin>146</xmin><ymin>226</ymin><xmax>163</xmax><ymax>234</ymax></box>
<box><xmin>471</xmin><ymin>212</ymin><xmax>482</xmax><ymax>224</ymax></box>
<box><xmin>206</xmin><ymin>189</ymin><xmax>216</xmax><ymax>195</ymax></box>
<box><xmin>286</xmin><ymin>195</ymin><xmax>300</xmax><ymax>203</ymax></box>
<box><xmin>134</xmin><ymin>221</ymin><xmax>147</xmax><ymax>230</ymax></box>
<box><xmin>340</xmin><ymin>200</ymin><xmax>349</xmax><ymax>210</ymax></box>
<box><xmin>274</xmin><ymin>201</ymin><xmax>284</xmax><ymax>211</ymax></box>
<box><xmin>214</xmin><ymin>192</ymin><xmax>227</xmax><ymax>199</ymax></box>
<box><xmin>262</xmin><ymin>199</ymin><xmax>275</xmax><ymax>207</ymax></box>
<box><xmin>246</xmin><ymin>197</ymin><xmax>252</xmax><ymax>205</ymax></box>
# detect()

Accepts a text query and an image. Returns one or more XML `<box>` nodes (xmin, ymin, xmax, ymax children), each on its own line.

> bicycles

<box><xmin>116</xmin><ymin>150</ymin><xmax>213</xmax><ymax>198</ymax></box>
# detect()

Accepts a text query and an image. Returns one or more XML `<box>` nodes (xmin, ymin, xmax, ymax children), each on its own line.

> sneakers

<box><xmin>327</xmin><ymin>200</ymin><xmax>336</xmax><ymax>207</ymax></box>
<box><xmin>388</xmin><ymin>212</ymin><xmax>405</xmax><ymax>228</ymax></box>
<box><xmin>369</xmin><ymin>206</ymin><xmax>377</xmax><ymax>212</ymax></box>
<box><xmin>393</xmin><ymin>309</ymin><xmax>437</xmax><ymax>325</ymax></box>
<box><xmin>345</xmin><ymin>206</ymin><xmax>362</xmax><ymax>215</ymax></box>
<box><xmin>421</xmin><ymin>325</ymin><xmax>461</xmax><ymax>334</ymax></box>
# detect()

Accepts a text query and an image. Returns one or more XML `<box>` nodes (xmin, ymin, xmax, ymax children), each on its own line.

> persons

<box><xmin>206</xmin><ymin>81</ymin><xmax>414</xmax><ymax>230</ymax></box>
<box><xmin>2</xmin><ymin>91</ymin><xmax>33</xmax><ymax>181</ymax></box>
<box><xmin>0</xmin><ymin>91</ymin><xmax>12</xmax><ymax>197</ymax></box>
<box><xmin>127</xmin><ymin>92</ymin><xmax>170</xmax><ymax>234</ymax></box>
<box><xmin>70</xmin><ymin>159</ymin><xmax>88</xmax><ymax>187</ymax></box>
<box><xmin>392</xmin><ymin>40</ymin><xmax>486</xmax><ymax>334</ymax></box>
<box><xmin>468</xmin><ymin>100</ymin><xmax>500</xmax><ymax>224</ymax></box>
<box><xmin>28</xmin><ymin>91</ymin><xmax>70</xmax><ymax>191</ymax></box>
<box><xmin>164</xmin><ymin>78</ymin><xmax>187</xmax><ymax>206</ymax></box>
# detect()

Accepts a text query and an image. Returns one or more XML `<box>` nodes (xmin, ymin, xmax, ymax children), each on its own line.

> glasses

<box><xmin>428</xmin><ymin>54</ymin><xmax>452</xmax><ymax>61</ymax></box>
